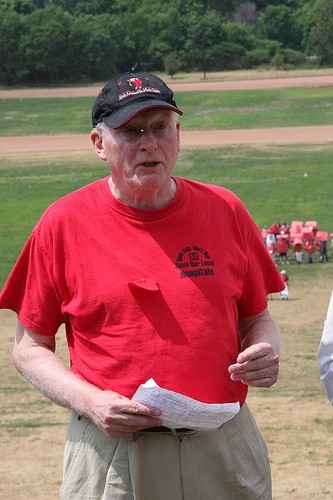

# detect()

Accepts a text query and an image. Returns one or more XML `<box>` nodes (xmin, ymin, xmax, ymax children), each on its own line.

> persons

<box><xmin>1</xmin><ymin>72</ymin><xmax>285</xmax><ymax>500</ymax></box>
<box><xmin>261</xmin><ymin>221</ymin><xmax>333</xmax><ymax>264</ymax></box>
<box><xmin>279</xmin><ymin>270</ymin><xmax>289</xmax><ymax>301</ymax></box>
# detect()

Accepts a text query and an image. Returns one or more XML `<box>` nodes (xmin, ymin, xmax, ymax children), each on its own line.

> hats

<box><xmin>92</xmin><ymin>70</ymin><xmax>183</xmax><ymax>129</ymax></box>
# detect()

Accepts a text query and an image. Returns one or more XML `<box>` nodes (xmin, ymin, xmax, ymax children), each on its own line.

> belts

<box><xmin>139</xmin><ymin>426</ymin><xmax>192</xmax><ymax>433</ymax></box>
